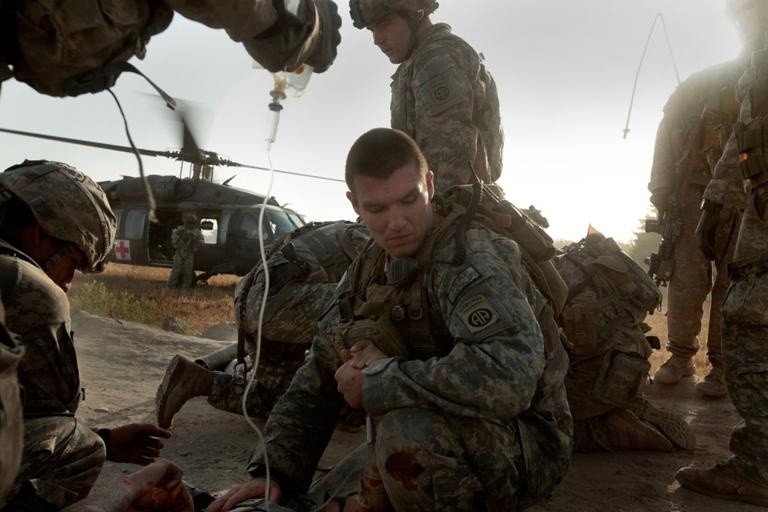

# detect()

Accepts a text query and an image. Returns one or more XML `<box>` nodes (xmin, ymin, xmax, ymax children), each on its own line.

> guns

<box><xmin>644</xmin><ymin>187</ymin><xmax>681</xmax><ymax>289</ymax></box>
<box><xmin>269</xmin><ymin>242</ymin><xmax>311</xmax><ymax>296</ymax></box>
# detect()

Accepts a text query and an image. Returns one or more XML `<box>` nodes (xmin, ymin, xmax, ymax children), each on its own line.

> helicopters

<box><xmin>0</xmin><ymin>60</ymin><xmax>348</xmax><ymax>287</ymax></box>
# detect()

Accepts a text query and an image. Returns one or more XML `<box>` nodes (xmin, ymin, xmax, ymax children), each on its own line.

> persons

<box><xmin>547</xmin><ymin>231</ymin><xmax>697</xmax><ymax>454</ymax></box>
<box><xmin>203</xmin><ymin>126</ymin><xmax>575</xmax><ymax>511</ymax></box>
<box><xmin>165</xmin><ymin>211</ymin><xmax>205</xmax><ymax>300</ymax></box>
<box><xmin>0</xmin><ymin>0</ymin><xmax>343</xmax><ymax>97</ymax></box>
<box><xmin>645</xmin><ymin>50</ymin><xmax>747</xmax><ymax>398</ymax></box>
<box><xmin>2</xmin><ymin>157</ymin><xmax>172</xmax><ymax>510</ymax></box>
<box><xmin>154</xmin><ymin>214</ymin><xmax>391</xmax><ymax>434</ymax></box>
<box><xmin>347</xmin><ymin>0</ymin><xmax>505</xmax><ymax>213</ymax></box>
<box><xmin>672</xmin><ymin>0</ymin><xmax>767</xmax><ymax>512</ymax></box>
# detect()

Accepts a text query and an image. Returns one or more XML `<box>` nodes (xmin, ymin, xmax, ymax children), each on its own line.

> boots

<box><xmin>588</xmin><ymin>395</ymin><xmax>696</xmax><ymax>454</ymax></box>
<box><xmin>696</xmin><ymin>368</ymin><xmax>727</xmax><ymax>396</ymax></box>
<box><xmin>654</xmin><ymin>348</ymin><xmax>696</xmax><ymax>384</ymax></box>
<box><xmin>675</xmin><ymin>457</ymin><xmax>768</xmax><ymax>505</ymax></box>
<box><xmin>155</xmin><ymin>354</ymin><xmax>214</xmax><ymax>430</ymax></box>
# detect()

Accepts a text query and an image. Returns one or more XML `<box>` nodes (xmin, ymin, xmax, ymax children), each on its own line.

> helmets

<box><xmin>0</xmin><ymin>159</ymin><xmax>117</xmax><ymax>273</ymax></box>
<box><xmin>349</xmin><ymin>0</ymin><xmax>438</xmax><ymax>30</ymax></box>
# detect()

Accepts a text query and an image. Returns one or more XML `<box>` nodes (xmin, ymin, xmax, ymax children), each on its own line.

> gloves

<box><xmin>245</xmin><ymin>0</ymin><xmax>341</xmax><ymax>75</ymax></box>
